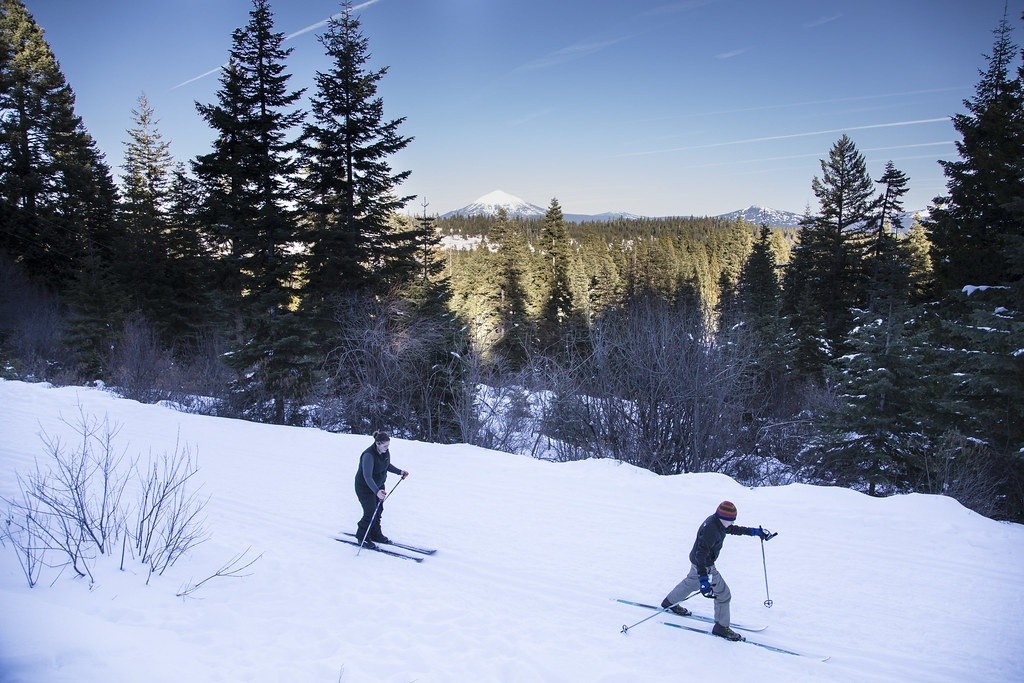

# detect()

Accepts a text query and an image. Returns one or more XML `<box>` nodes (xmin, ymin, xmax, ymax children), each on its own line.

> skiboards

<box><xmin>330</xmin><ymin>527</ymin><xmax>437</xmax><ymax>563</ymax></box>
<box><xmin>606</xmin><ymin>595</ymin><xmax>829</xmax><ymax>664</ymax></box>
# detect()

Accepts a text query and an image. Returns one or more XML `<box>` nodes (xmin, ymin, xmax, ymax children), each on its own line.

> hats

<box><xmin>716</xmin><ymin>501</ymin><xmax>737</xmax><ymax>521</ymax></box>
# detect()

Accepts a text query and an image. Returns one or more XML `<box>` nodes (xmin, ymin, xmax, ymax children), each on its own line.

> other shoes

<box><xmin>371</xmin><ymin>535</ymin><xmax>388</xmax><ymax>542</ymax></box>
<box><xmin>358</xmin><ymin>539</ymin><xmax>376</xmax><ymax>549</ymax></box>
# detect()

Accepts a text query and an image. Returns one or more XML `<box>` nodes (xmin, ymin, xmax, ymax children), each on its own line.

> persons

<box><xmin>355</xmin><ymin>432</ymin><xmax>409</xmax><ymax>548</ymax></box>
<box><xmin>661</xmin><ymin>501</ymin><xmax>773</xmax><ymax>641</ymax></box>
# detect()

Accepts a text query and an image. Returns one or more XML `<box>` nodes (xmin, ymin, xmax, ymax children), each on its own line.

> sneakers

<box><xmin>660</xmin><ymin>596</ymin><xmax>692</xmax><ymax>616</ymax></box>
<box><xmin>712</xmin><ymin>624</ymin><xmax>742</xmax><ymax>641</ymax></box>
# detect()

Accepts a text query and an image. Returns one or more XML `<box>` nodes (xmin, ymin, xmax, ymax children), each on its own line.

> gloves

<box><xmin>698</xmin><ymin>575</ymin><xmax>717</xmax><ymax>598</ymax></box>
<box><xmin>751</xmin><ymin>527</ymin><xmax>778</xmax><ymax>541</ymax></box>
